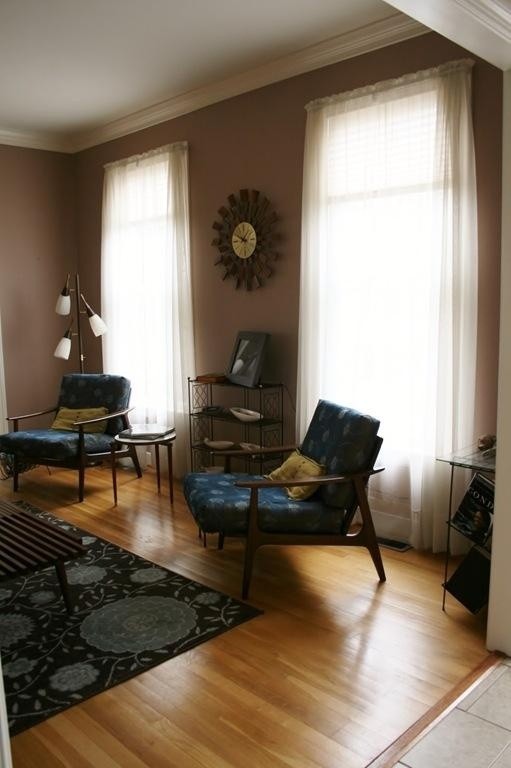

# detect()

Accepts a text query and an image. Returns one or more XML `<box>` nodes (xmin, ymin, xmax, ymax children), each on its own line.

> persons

<box><xmin>458</xmin><ymin>509</ymin><xmax>493</xmax><ymax>544</ymax></box>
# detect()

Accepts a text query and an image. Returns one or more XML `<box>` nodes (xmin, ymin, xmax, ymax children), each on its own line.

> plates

<box><xmin>202</xmin><ymin>405</ymin><xmax>265</xmax><ymax>474</ymax></box>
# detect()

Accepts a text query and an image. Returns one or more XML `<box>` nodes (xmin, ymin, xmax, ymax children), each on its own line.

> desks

<box><xmin>109</xmin><ymin>424</ymin><xmax>176</xmax><ymax>504</ymax></box>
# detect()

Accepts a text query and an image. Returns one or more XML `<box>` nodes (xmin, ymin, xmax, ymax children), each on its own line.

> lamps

<box><xmin>54</xmin><ymin>271</ymin><xmax>105</xmax><ymax>470</ymax></box>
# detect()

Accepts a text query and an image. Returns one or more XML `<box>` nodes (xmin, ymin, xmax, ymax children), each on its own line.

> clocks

<box><xmin>208</xmin><ymin>188</ymin><xmax>285</xmax><ymax>292</ymax></box>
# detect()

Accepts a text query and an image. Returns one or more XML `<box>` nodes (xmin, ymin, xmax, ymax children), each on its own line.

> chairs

<box><xmin>1</xmin><ymin>373</ymin><xmax>141</xmax><ymax>503</ymax></box>
<box><xmin>181</xmin><ymin>400</ymin><xmax>387</xmax><ymax>601</ymax></box>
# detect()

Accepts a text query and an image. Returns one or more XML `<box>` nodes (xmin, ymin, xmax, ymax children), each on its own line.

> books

<box><xmin>195</xmin><ymin>370</ymin><xmax>226</xmax><ymax>383</ymax></box>
<box><xmin>117</xmin><ymin>425</ymin><xmax>175</xmax><ymax>440</ymax></box>
<box><xmin>450</xmin><ymin>470</ymin><xmax>495</xmax><ymax>553</ymax></box>
<box><xmin>443</xmin><ymin>544</ymin><xmax>491</xmax><ymax>616</ymax></box>
<box><xmin>130</xmin><ymin>422</ymin><xmax>165</xmax><ymax>436</ymax></box>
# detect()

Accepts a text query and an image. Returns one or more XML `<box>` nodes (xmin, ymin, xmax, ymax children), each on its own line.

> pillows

<box><xmin>264</xmin><ymin>446</ymin><xmax>327</xmax><ymax>502</ymax></box>
<box><xmin>46</xmin><ymin>406</ymin><xmax>110</xmax><ymax>437</ymax></box>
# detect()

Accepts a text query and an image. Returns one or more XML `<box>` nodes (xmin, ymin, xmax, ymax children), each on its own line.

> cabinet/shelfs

<box><xmin>188</xmin><ymin>375</ymin><xmax>285</xmax><ymax>476</ymax></box>
<box><xmin>434</xmin><ymin>439</ymin><xmax>494</xmax><ymax>613</ymax></box>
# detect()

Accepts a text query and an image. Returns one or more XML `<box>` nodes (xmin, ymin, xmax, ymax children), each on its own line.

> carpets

<box><xmin>0</xmin><ymin>484</ymin><xmax>266</xmax><ymax>739</ymax></box>
